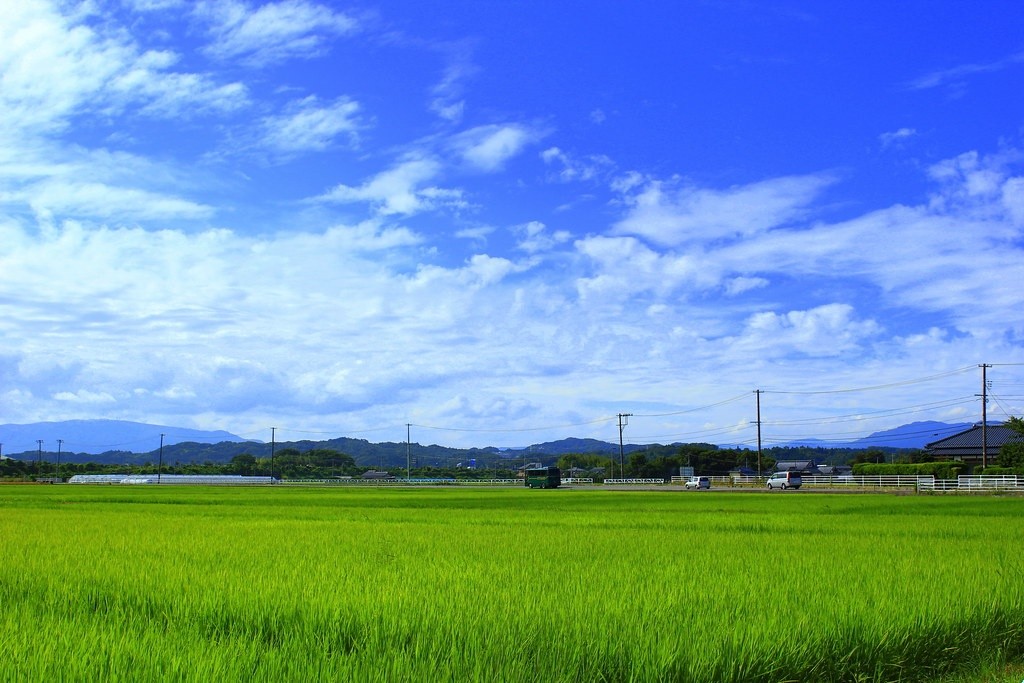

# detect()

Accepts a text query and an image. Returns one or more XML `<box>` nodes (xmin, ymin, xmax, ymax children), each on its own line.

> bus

<box><xmin>523</xmin><ymin>466</ymin><xmax>562</xmax><ymax>489</ymax></box>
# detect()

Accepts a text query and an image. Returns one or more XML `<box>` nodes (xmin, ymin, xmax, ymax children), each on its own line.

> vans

<box><xmin>767</xmin><ymin>471</ymin><xmax>802</xmax><ymax>490</ymax></box>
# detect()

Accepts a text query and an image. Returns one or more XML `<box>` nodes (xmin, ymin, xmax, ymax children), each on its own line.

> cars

<box><xmin>684</xmin><ymin>476</ymin><xmax>711</xmax><ymax>489</ymax></box>
